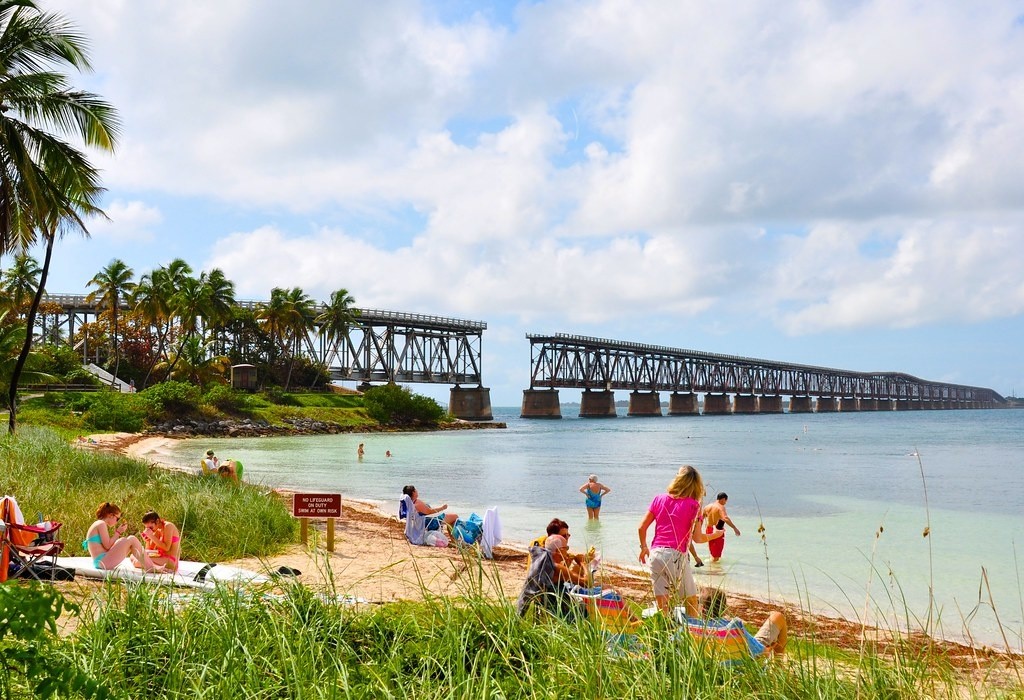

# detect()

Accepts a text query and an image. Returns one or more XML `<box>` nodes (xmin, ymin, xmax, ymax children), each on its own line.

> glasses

<box><xmin>114</xmin><ymin>515</ymin><xmax>120</xmax><ymax>521</ymax></box>
<box><xmin>561</xmin><ymin>546</ymin><xmax>570</xmax><ymax>550</ymax></box>
<box><xmin>562</xmin><ymin>534</ymin><xmax>570</xmax><ymax>538</ymax></box>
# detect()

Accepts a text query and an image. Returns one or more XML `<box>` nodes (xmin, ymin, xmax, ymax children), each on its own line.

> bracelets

<box><xmin>641</xmin><ymin>545</ymin><xmax>647</xmax><ymax>548</ymax></box>
<box><xmin>706</xmin><ymin>535</ymin><xmax>711</xmax><ymax>542</ymax></box>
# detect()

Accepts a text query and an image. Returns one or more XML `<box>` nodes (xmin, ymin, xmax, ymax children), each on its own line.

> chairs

<box><xmin>562</xmin><ymin>582</ymin><xmax>650</xmax><ymax>661</ymax></box>
<box><xmin>400</xmin><ymin>494</ymin><xmax>446</xmax><ymax>547</ymax></box>
<box><xmin>4</xmin><ymin>498</ymin><xmax>65</xmax><ymax>584</ymax></box>
<box><xmin>455</xmin><ymin>505</ymin><xmax>498</xmax><ymax>559</ymax></box>
<box><xmin>201</xmin><ymin>459</ymin><xmax>219</xmax><ymax>477</ymax></box>
<box><xmin>683</xmin><ymin>614</ymin><xmax>770</xmax><ymax>674</ymax></box>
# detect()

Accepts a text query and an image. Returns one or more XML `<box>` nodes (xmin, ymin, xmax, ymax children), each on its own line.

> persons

<box><xmin>83</xmin><ymin>503</ymin><xmax>167</xmax><ymax>573</ymax></box>
<box><xmin>671</xmin><ymin>588</ymin><xmax>788</xmax><ymax>666</ymax></box>
<box><xmin>129</xmin><ymin>378</ymin><xmax>135</xmax><ymax>392</ymax></box>
<box><xmin>77</xmin><ymin>436</ymin><xmax>97</xmax><ymax>444</ymax></box>
<box><xmin>689</xmin><ymin>542</ymin><xmax>705</xmax><ymax>567</ymax></box>
<box><xmin>386</xmin><ymin>451</ymin><xmax>391</xmax><ymax>456</ymax></box>
<box><xmin>702</xmin><ymin>492</ymin><xmax>740</xmax><ymax>560</ymax></box>
<box><xmin>203</xmin><ymin>449</ymin><xmax>218</xmax><ymax>472</ymax></box>
<box><xmin>515</xmin><ymin>519</ymin><xmax>595</xmax><ymax>616</ymax></box>
<box><xmin>219</xmin><ymin>461</ymin><xmax>243</xmax><ymax>482</ymax></box>
<box><xmin>358</xmin><ymin>444</ymin><xmax>366</xmax><ymax>455</ymax></box>
<box><xmin>579</xmin><ymin>474</ymin><xmax>611</xmax><ymax>519</ymax></box>
<box><xmin>131</xmin><ymin>509</ymin><xmax>180</xmax><ymax>572</ymax></box>
<box><xmin>639</xmin><ymin>466</ymin><xmax>725</xmax><ymax>618</ymax></box>
<box><xmin>403</xmin><ymin>485</ymin><xmax>459</xmax><ymax>542</ymax></box>
